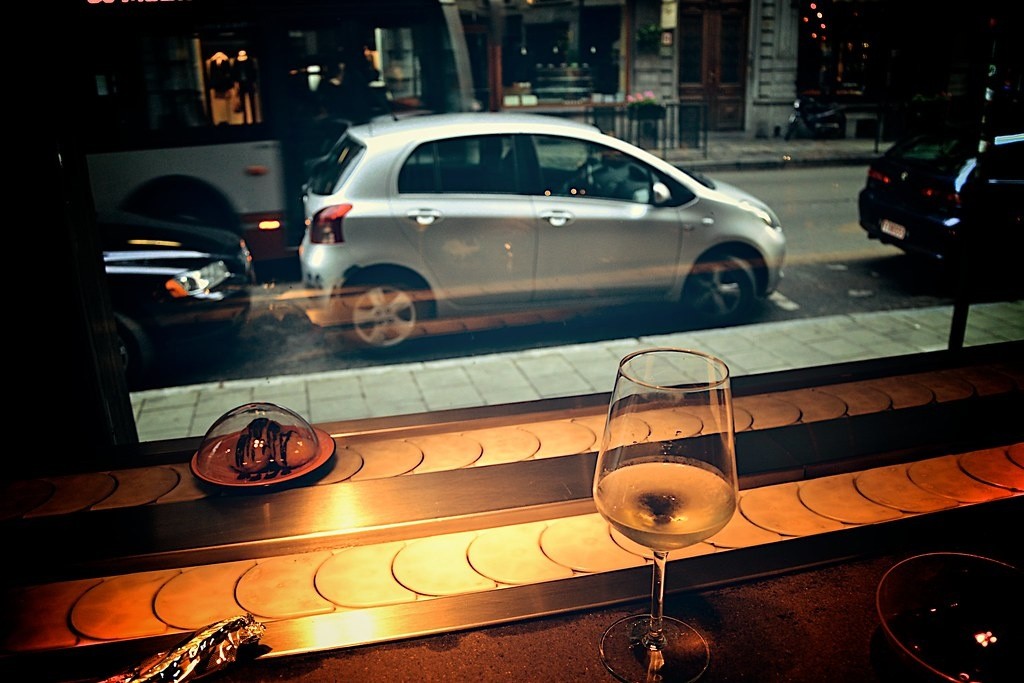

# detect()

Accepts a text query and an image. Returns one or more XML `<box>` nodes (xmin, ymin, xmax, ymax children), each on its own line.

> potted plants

<box><xmin>634</xmin><ymin>22</ymin><xmax>663</xmax><ymax>57</ymax></box>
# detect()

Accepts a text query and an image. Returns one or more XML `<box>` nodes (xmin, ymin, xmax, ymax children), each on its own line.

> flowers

<box><xmin>627</xmin><ymin>91</ymin><xmax>665</xmax><ymax>112</ymax></box>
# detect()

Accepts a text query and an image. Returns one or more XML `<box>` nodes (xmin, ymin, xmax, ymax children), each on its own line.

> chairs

<box><xmin>502</xmin><ymin>149</ymin><xmax>518</xmax><ymax>195</ymax></box>
<box><xmin>476</xmin><ymin>139</ymin><xmax>505</xmax><ymax>191</ymax></box>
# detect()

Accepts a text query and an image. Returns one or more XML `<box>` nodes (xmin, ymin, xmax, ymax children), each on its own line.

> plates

<box><xmin>876</xmin><ymin>552</ymin><xmax>1024</xmax><ymax>683</ymax></box>
<box><xmin>189</xmin><ymin>426</ymin><xmax>335</xmax><ymax>488</ymax></box>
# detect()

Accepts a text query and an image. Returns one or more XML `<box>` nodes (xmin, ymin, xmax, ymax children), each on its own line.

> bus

<box><xmin>72</xmin><ymin>1</ymin><xmax>524</xmax><ymax>256</ymax></box>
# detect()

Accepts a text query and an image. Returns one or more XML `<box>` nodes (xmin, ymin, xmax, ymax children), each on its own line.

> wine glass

<box><xmin>592</xmin><ymin>348</ymin><xmax>738</xmax><ymax>683</ymax></box>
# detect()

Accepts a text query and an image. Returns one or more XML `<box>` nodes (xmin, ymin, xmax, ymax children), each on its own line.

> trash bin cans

<box><xmin>593</xmin><ymin>106</ymin><xmax>614</xmax><ymax>133</ymax></box>
<box><xmin>640</xmin><ymin>119</ymin><xmax>658</xmax><ymax>149</ymax></box>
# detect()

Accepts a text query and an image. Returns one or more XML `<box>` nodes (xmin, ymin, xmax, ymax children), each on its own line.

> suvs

<box><xmin>858</xmin><ymin>98</ymin><xmax>1024</xmax><ymax>287</ymax></box>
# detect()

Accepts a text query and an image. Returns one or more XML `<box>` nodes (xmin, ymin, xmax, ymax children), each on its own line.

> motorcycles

<box><xmin>784</xmin><ymin>81</ymin><xmax>853</xmax><ymax>141</ymax></box>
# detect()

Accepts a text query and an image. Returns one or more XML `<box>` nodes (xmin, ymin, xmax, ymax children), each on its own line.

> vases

<box><xmin>626</xmin><ymin>109</ymin><xmax>667</xmax><ymax>122</ymax></box>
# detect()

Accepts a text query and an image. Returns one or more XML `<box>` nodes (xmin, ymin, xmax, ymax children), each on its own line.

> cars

<box><xmin>299</xmin><ymin>113</ymin><xmax>785</xmax><ymax>358</ymax></box>
<box><xmin>93</xmin><ymin>203</ymin><xmax>252</xmax><ymax>392</ymax></box>
